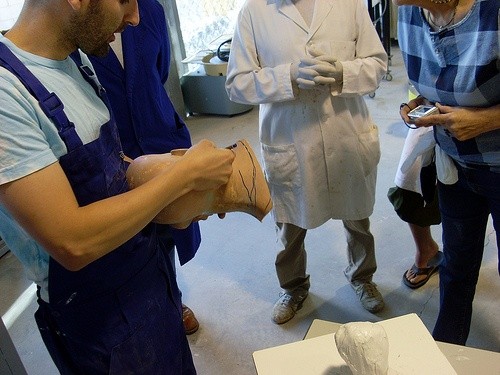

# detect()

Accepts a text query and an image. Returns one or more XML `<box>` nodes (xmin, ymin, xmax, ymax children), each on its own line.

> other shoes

<box><xmin>181</xmin><ymin>303</ymin><xmax>200</xmax><ymax>336</ymax></box>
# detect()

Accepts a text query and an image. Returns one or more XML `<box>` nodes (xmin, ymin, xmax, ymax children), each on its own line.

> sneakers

<box><xmin>270</xmin><ymin>290</ymin><xmax>310</xmax><ymax>323</ymax></box>
<box><xmin>351</xmin><ymin>278</ymin><xmax>385</xmax><ymax>312</ymax></box>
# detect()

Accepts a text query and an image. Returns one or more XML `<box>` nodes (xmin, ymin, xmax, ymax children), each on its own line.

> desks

<box><xmin>301</xmin><ymin>319</ymin><xmax>500</xmax><ymax>375</ymax></box>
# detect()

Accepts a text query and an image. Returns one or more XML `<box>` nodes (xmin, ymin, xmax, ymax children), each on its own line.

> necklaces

<box><xmin>428</xmin><ymin>5</ymin><xmax>458</xmax><ymax>28</ymax></box>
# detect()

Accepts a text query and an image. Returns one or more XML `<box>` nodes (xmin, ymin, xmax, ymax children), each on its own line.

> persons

<box><xmin>226</xmin><ymin>0</ymin><xmax>388</xmax><ymax>323</ymax></box>
<box><xmin>388</xmin><ymin>80</ymin><xmax>445</xmax><ymax>288</ymax></box>
<box><xmin>393</xmin><ymin>0</ymin><xmax>500</xmax><ymax>347</ymax></box>
<box><xmin>0</xmin><ymin>0</ymin><xmax>235</xmax><ymax>375</ymax></box>
<box><xmin>87</xmin><ymin>0</ymin><xmax>202</xmax><ymax>335</ymax></box>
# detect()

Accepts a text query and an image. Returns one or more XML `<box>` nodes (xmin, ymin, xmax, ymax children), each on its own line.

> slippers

<box><xmin>400</xmin><ymin>250</ymin><xmax>443</xmax><ymax>290</ymax></box>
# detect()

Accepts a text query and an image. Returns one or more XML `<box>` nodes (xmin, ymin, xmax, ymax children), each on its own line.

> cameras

<box><xmin>407</xmin><ymin>105</ymin><xmax>440</xmax><ymax>124</ymax></box>
